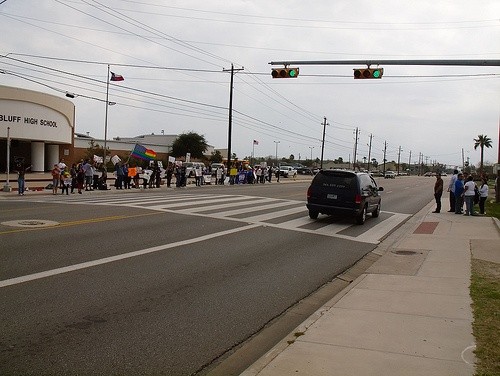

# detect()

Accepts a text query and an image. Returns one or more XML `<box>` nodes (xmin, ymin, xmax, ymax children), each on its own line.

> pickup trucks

<box><xmin>275</xmin><ymin>166</ymin><xmax>298</xmax><ymax>179</ymax></box>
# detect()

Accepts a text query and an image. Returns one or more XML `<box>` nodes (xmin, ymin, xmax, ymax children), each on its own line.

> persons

<box><xmin>455</xmin><ymin>174</ymin><xmax>464</xmax><ymax>214</ymax></box>
<box><xmin>479</xmin><ymin>178</ymin><xmax>488</xmax><ymax>215</ymax></box>
<box><xmin>461</xmin><ymin>176</ymin><xmax>478</xmax><ymax>216</ymax></box>
<box><xmin>52</xmin><ymin>155</ymin><xmax>280</xmax><ymax>196</ymax></box>
<box><xmin>18</xmin><ymin>163</ymin><xmax>32</xmax><ymax>196</ymax></box>
<box><xmin>495</xmin><ymin>176</ymin><xmax>499</xmax><ymax>197</ymax></box>
<box><xmin>463</xmin><ymin>172</ymin><xmax>468</xmax><ymax>184</ymax></box>
<box><xmin>447</xmin><ymin>170</ymin><xmax>458</xmax><ymax>212</ymax></box>
<box><xmin>294</xmin><ymin>172</ymin><xmax>296</xmax><ymax>181</ymax></box>
<box><xmin>432</xmin><ymin>173</ymin><xmax>443</xmax><ymax>213</ymax></box>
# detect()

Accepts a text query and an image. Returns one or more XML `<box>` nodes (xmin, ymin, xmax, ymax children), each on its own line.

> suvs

<box><xmin>306</xmin><ymin>168</ymin><xmax>385</xmax><ymax>226</ymax></box>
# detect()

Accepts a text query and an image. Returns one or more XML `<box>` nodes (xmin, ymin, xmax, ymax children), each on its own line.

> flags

<box><xmin>254</xmin><ymin>140</ymin><xmax>258</xmax><ymax>144</ymax></box>
<box><xmin>131</xmin><ymin>143</ymin><xmax>156</xmax><ymax>160</ymax></box>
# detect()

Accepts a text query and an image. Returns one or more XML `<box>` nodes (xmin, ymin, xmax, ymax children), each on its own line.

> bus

<box><xmin>223</xmin><ymin>159</ymin><xmax>250</xmax><ymax>172</ymax></box>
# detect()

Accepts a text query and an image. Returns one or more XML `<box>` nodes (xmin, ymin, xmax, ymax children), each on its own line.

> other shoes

<box><xmin>432</xmin><ymin>210</ymin><xmax>440</xmax><ymax>213</ymax></box>
<box><xmin>116</xmin><ymin>188</ymin><xmax>119</xmax><ymax>190</ymax></box>
<box><xmin>448</xmin><ymin>210</ymin><xmax>456</xmax><ymax>212</ymax></box>
<box><xmin>455</xmin><ymin>212</ymin><xmax>464</xmax><ymax>214</ymax></box>
<box><xmin>464</xmin><ymin>214</ymin><xmax>469</xmax><ymax>216</ymax></box>
<box><xmin>71</xmin><ymin>191</ymin><xmax>75</xmax><ymax>194</ymax></box>
<box><xmin>119</xmin><ymin>188</ymin><xmax>124</xmax><ymax>190</ymax></box>
<box><xmin>90</xmin><ymin>189</ymin><xmax>94</xmax><ymax>191</ymax></box>
<box><xmin>18</xmin><ymin>193</ymin><xmax>24</xmax><ymax>196</ymax></box>
<box><xmin>85</xmin><ymin>189</ymin><xmax>90</xmax><ymax>191</ymax></box>
<box><xmin>78</xmin><ymin>191</ymin><xmax>82</xmax><ymax>194</ymax></box>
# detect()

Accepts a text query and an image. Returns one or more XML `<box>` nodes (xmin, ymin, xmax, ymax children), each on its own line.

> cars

<box><xmin>182</xmin><ymin>162</ymin><xmax>207</xmax><ymax>178</ymax></box>
<box><xmin>211</xmin><ymin>163</ymin><xmax>225</xmax><ymax>178</ymax></box>
<box><xmin>254</xmin><ymin>162</ymin><xmax>321</xmax><ymax>176</ymax></box>
<box><xmin>384</xmin><ymin>170</ymin><xmax>397</xmax><ymax>179</ymax></box>
<box><xmin>424</xmin><ymin>171</ymin><xmax>447</xmax><ymax>178</ymax></box>
<box><xmin>363</xmin><ymin>170</ymin><xmax>409</xmax><ymax>177</ymax></box>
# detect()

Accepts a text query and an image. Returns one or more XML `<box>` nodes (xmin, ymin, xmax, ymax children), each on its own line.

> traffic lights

<box><xmin>271</xmin><ymin>67</ymin><xmax>300</xmax><ymax>79</ymax></box>
<box><xmin>353</xmin><ymin>68</ymin><xmax>384</xmax><ymax>79</ymax></box>
